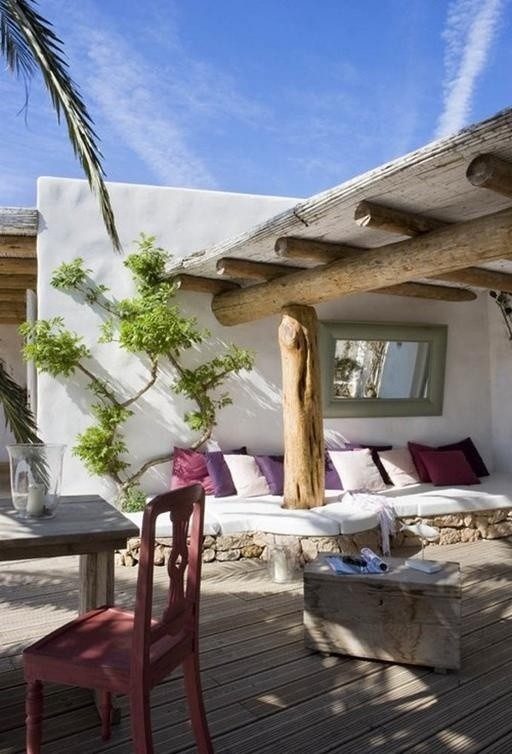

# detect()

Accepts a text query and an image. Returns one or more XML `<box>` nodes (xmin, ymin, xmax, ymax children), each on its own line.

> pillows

<box><xmin>323</xmin><ymin>436</ymin><xmax>491</xmax><ymax>492</ymax></box>
<box><xmin>168</xmin><ymin>444</ymin><xmax>286</xmax><ymax>497</ymax></box>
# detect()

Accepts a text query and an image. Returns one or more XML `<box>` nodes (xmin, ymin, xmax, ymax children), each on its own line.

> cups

<box><xmin>264</xmin><ymin>540</ymin><xmax>298</xmax><ymax>584</ymax></box>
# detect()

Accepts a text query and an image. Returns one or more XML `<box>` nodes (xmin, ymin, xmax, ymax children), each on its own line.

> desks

<box><xmin>0</xmin><ymin>491</ymin><xmax>136</xmax><ymax>726</ymax></box>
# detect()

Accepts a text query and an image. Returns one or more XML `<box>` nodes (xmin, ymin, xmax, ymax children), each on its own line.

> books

<box><xmin>404</xmin><ymin>555</ymin><xmax>442</xmax><ymax>573</ymax></box>
<box><xmin>325</xmin><ymin>548</ymin><xmax>388</xmax><ymax>576</ymax></box>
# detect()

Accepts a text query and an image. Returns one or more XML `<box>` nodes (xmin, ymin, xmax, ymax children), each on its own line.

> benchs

<box><xmin>117</xmin><ymin>471</ymin><xmax>512</xmax><ymax>563</ymax></box>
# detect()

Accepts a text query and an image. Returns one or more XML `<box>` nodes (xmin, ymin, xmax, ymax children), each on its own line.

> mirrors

<box><xmin>318</xmin><ymin>317</ymin><xmax>447</xmax><ymax>418</ymax></box>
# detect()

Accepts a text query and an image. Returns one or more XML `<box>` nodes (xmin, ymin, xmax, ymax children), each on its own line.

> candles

<box><xmin>25</xmin><ymin>483</ymin><xmax>45</xmax><ymax>516</ymax></box>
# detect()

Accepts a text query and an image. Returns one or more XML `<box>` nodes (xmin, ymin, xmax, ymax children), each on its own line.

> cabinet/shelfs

<box><xmin>303</xmin><ymin>553</ymin><xmax>463</xmax><ymax>676</ymax></box>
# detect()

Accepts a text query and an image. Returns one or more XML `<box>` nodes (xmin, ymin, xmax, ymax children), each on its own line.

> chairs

<box><xmin>15</xmin><ymin>483</ymin><xmax>212</xmax><ymax>754</ymax></box>
